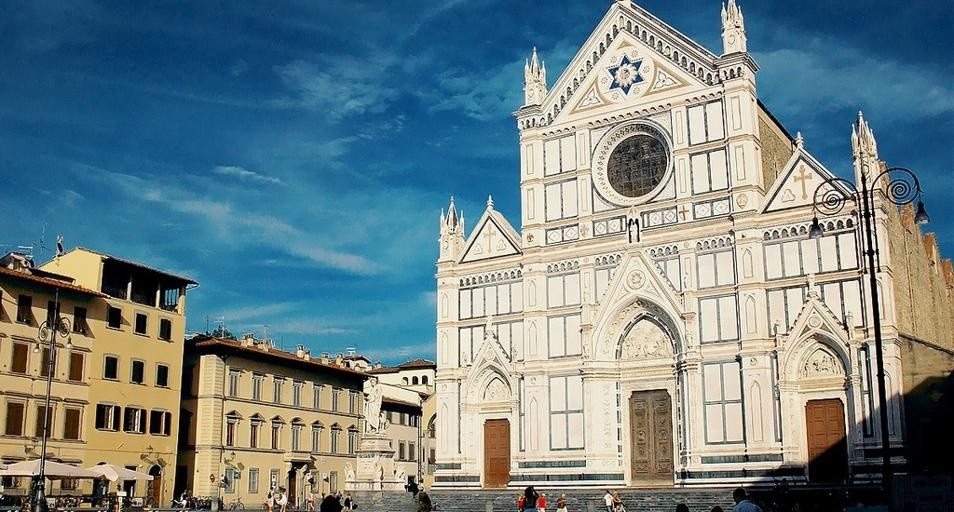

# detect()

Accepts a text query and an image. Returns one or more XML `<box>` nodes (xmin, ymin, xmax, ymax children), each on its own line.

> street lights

<box><xmin>31</xmin><ymin>286</ymin><xmax>74</xmax><ymax>512</ymax></box>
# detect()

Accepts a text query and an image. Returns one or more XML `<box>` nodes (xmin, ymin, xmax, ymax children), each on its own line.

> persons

<box><xmin>369</xmin><ymin>455</ymin><xmax>383</xmax><ymax>480</ymax></box>
<box><xmin>363</xmin><ymin>377</ymin><xmax>390</xmax><ymax>434</ymax></box>
<box><xmin>344</xmin><ymin>461</ymin><xmax>355</xmax><ymax>481</ymax></box>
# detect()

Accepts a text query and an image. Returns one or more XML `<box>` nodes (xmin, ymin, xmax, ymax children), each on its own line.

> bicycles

<box><xmin>196</xmin><ymin>495</ymin><xmax>226</xmax><ymax>512</ymax></box>
<box><xmin>228</xmin><ymin>497</ymin><xmax>244</xmax><ymax>512</ymax></box>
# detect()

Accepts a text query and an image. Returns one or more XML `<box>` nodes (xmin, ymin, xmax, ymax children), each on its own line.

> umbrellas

<box><xmin>0</xmin><ymin>458</ymin><xmax>154</xmax><ymax>483</ymax></box>
